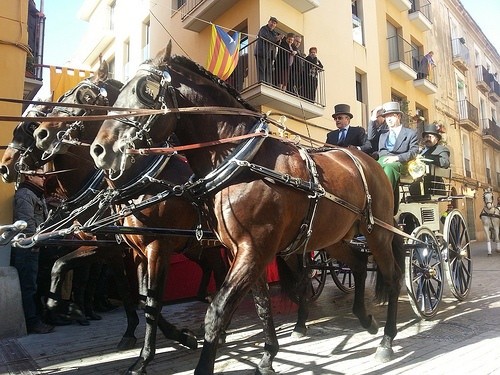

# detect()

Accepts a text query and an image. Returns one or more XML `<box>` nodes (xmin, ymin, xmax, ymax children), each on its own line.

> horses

<box><xmin>0</xmin><ymin>91</ymin><xmax>227</xmax><ymax>350</ymax></box>
<box><xmin>33</xmin><ymin>59</ymin><xmax>313</xmax><ymax>375</ymax></box>
<box><xmin>90</xmin><ymin>39</ymin><xmax>406</xmax><ymax>375</ymax></box>
<box><xmin>479</xmin><ymin>186</ymin><xmax>500</xmax><ymax>255</ymax></box>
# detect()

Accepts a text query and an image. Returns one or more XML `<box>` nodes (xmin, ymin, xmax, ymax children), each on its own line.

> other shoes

<box><xmin>28</xmin><ymin>323</ymin><xmax>55</xmax><ymax>334</ymax></box>
<box><xmin>71</xmin><ymin>307</ymin><xmax>101</xmax><ymax>326</ymax></box>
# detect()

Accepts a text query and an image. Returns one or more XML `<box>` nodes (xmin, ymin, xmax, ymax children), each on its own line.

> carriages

<box><xmin>0</xmin><ymin>39</ymin><xmax>473</xmax><ymax>375</ymax></box>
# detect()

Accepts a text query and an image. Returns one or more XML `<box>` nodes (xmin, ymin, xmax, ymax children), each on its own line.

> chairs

<box><xmin>398</xmin><ymin>159</ymin><xmax>454</xmax><ymax>202</ymax></box>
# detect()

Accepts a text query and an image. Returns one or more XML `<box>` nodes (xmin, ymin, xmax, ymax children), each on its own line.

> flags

<box><xmin>205</xmin><ymin>24</ymin><xmax>242</xmax><ymax>81</ymax></box>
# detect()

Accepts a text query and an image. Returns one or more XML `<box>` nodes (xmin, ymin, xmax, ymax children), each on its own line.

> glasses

<box><xmin>334</xmin><ymin>117</ymin><xmax>347</xmax><ymax>120</ymax></box>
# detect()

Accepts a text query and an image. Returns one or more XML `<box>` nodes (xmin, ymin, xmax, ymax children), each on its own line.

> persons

<box><xmin>409</xmin><ymin>124</ymin><xmax>451</xmax><ymax>195</ymax></box>
<box><xmin>323</xmin><ymin>104</ymin><xmax>372</xmax><ymax>151</ymax></box>
<box><xmin>418</xmin><ymin>51</ymin><xmax>434</xmax><ymax>79</ymax></box>
<box><xmin>10</xmin><ymin>165</ymin><xmax>120</xmax><ymax>334</ymax></box>
<box><xmin>376</xmin><ymin>101</ymin><xmax>419</xmax><ymax>192</ymax></box>
<box><xmin>254</xmin><ymin>17</ymin><xmax>323</xmax><ymax>103</ymax></box>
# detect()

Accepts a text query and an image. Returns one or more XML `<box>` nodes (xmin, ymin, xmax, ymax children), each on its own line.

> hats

<box><xmin>381</xmin><ymin>102</ymin><xmax>404</xmax><ymax>118</ymax></box>
<box><xmin>332</xmin><ymin>104</ymin><xmax>353</xmax><ymax>119</ymax></box>
<box><xmin>376</xmin><ymin>110</ymin><xmax>381</xmax><ymax>118</ymax></box>
<box><xmin>422</xmin><ymin>124</ymin><xmax>442</xmax><ymax>140</ymax></box>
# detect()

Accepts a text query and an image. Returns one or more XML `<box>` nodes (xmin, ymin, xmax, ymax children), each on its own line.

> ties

<box><xmin>340</xmin><ymin>129</ymin><xmax>347</xmax><ymax>143</ymax></box>
